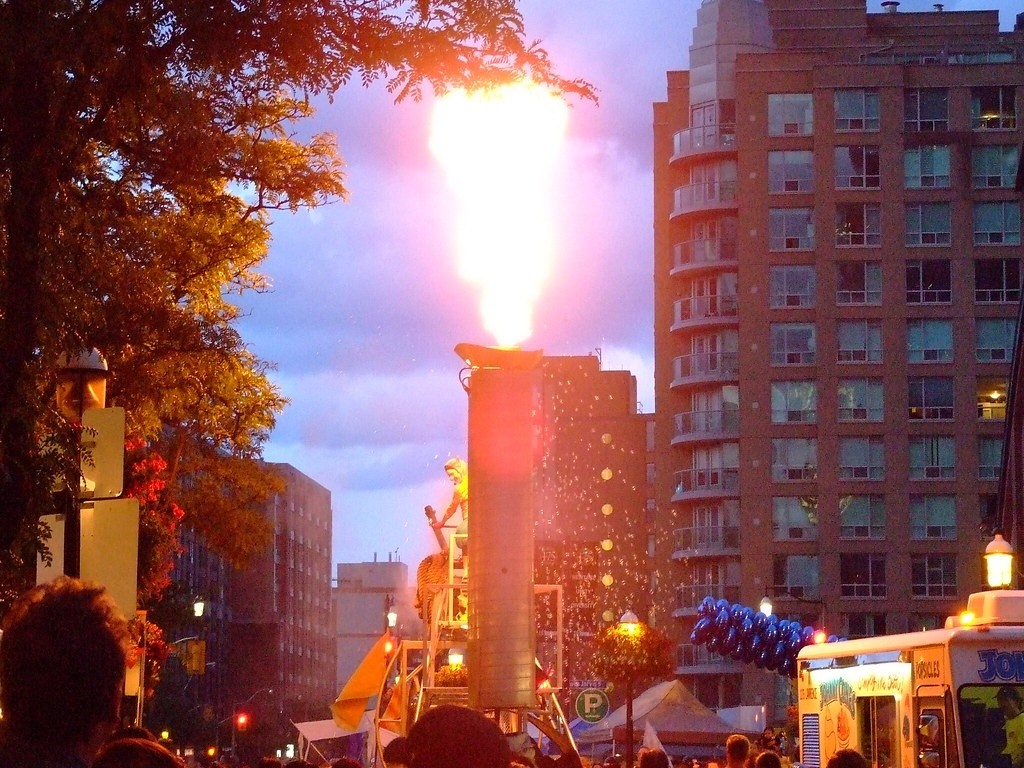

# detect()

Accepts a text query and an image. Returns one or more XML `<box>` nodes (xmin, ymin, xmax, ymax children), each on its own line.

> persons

<box><xmin>256</xmin><ymin>705</ymin><xmax>512</xmax><ymax>768</ymax></box>
<box><xmin>992</xmin><ymin>686</ymin><xmax>1024</xmax><ymax>768</ymax></box>
<box><xmin>723</xmin><ymin>727</ymin><xmax>782</xmax><ymax>768</ymax></box>
<box><xmin>603</xmin><ymin>748</ymin><xmax>694</xmax><ymax>768</ymax></box>
<box><xmin>820</xmin><ymin>749</ymin><xmax>869</xmax><ymax>768</ymax></box>
<box><xmin>432</xmin><ymin>458</ymin><xmax>468</xmax><ymax>577</ymax></box>
<box><xmin>0</xmin><ymin>575</ymin><xmax>186</xmax><ymax>768</ymax></box>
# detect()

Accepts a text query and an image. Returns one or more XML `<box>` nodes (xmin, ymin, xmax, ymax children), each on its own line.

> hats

<box><xmin>992</xmin><ymin>687</ymin><xmax>1022</xmax><ymax>701</ymax></box>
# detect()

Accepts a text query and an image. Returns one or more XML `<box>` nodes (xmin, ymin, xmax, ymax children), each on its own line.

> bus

<box><xmin>797</xmin><ymin>626</ymin><xmax>1024</xmax><ymax>768</ymax></box>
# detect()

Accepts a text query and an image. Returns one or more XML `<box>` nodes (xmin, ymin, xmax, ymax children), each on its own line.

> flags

<box><xmin>329</xmin><ymin>632</ymin><xmax>386</xmax><ymax>732</ymax></box>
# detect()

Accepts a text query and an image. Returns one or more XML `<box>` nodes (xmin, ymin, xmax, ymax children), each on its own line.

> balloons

<box><xmin>690</xmin><ymin>596</ymin><xmax>848</xmax><ymax>679</ymax></box>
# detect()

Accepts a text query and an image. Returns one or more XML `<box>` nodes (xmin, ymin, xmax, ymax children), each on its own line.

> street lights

<box><xmin>381</xmin><ymin>591</ymin><xmax>405</xmax><ymax>697</ymax></box>
<box><xmin>616</xmin><ymin>608</ymin><xmax>641</xmax><ymax>768</ymax></box>
<box><xmin>220</xmin><ymin>711</ymin><xmax>247</xmax><ymax>763</ymax></box>
<box><xmin>192</xmin><ymin>586</ymin><xmax>222</xmax><ymax>715</ymax></box>
<box><xmin>55</xmin><ymin>344</ymin><xmax>109</xmax><ymax>576</ymax></box>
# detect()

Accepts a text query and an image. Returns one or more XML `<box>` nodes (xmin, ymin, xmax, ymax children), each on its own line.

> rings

<box><xmin>433</xmin><ymin>524</ymin><xmax>434</xmax><ymax>526</ymax></box>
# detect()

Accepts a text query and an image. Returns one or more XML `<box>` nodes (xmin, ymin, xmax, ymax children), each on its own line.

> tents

<box><xmin>541</xmin><ymin>679</ymin><xmax>762</xmax><ymax>758</ymax></box>
